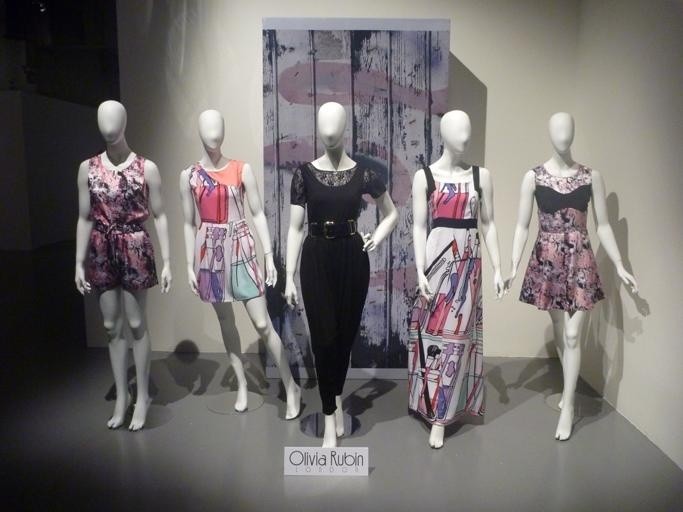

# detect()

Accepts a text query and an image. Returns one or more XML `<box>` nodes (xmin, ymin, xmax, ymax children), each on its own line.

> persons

<box><xmin>178</xmin><ymin>109</ymin><xmax>303</xmax><ymax>422</ymax></box>
<box><xmin>504</xmin><ymin>112</ymin><xmax>638</xmax><ymax>440</ymax></box>
<box><xmin>282</xmin><ymin>101</ymin><xmax>401</xmax><ymax>448</ymax></box>
<box><xmin>74</xmin><ymin>99</ymin><xmax>173</xmax><ymax>431</ymax></box>
<box><xmin>407</xmin><ymin>109</ymin><xmax>504</xmax><ymax>448</ymax></box>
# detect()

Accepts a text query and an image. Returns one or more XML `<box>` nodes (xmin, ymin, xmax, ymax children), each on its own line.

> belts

<box><xmin>308</xmin><ymin>220</ymin><xmax>357</xmax><ymax>239</ymax></box>
